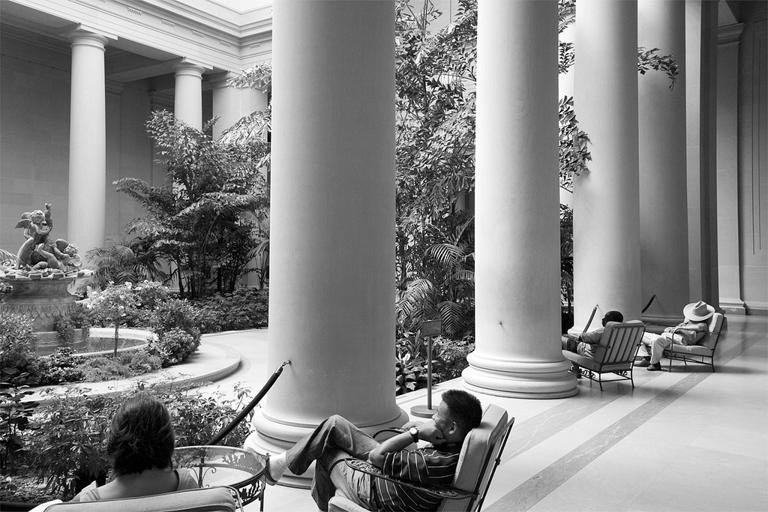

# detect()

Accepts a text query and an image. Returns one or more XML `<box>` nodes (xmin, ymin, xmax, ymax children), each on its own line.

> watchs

<box><xmin>409</xmin><ymin>427</ymin><xmax>419</xmax><ymax>443</ymax></box>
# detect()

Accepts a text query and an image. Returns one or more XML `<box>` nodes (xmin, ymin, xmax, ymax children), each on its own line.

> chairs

<box><xmin>562</xmin><ymin>311</ymin><xmax>725</xmax><ymax>392</ymax></box>
<box><xmin>44</xmin><ymin>485</ymin><xmax>243</xmax><ymax>511</ymax></box>
<box><xmin>323</xmin><ymin>403</ymin><xmax>515</xmax><ymax>512</ymax></box>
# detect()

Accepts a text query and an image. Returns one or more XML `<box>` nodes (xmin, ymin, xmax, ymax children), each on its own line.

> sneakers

<box><xmin>568</xmin><ymin>365</ymin><xmax>582</xmax><ymax>378</ymax></box>
<box><xmin>245</xmin><ymin>447</ymin><xmax>279</xmax><ymax>487</ymax></box>
<box><xmin>633</xmin><ymin>358</ymin><xmax>651</xmax><ymax>367</ymax></box>
<box><xmin>647</xmin><ymin>364</ymin><xmax>662</xmax><ymax>371</ymax></box>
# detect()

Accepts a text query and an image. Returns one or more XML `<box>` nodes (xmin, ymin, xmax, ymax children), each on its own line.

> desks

<box><xmin>171</xmin><ymin>444</ymin><xmax>268</xmax><ymax>510</ymax></box>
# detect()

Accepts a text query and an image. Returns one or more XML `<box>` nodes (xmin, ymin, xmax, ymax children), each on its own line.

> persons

<box><xmin>14</xmin><ymin>202</ymin><xmax>77</xmax><ymax>272</ymax></box>
<box><xmin>633</xmin><ymin>300</ymin><xmax>716</xmax><ymax>371</ymax></box>
<box><xmin>68</xmin><ymin>395</ymin><xmax>200</xmax><ymax>502</ymax></box>
<box><xmin>561</xmin><ymin>311</ymin><xmax>624</xmax><ymax>378</ymax></box>
<box><xmin>245</xmin><ymin>389</ymin><xmax>482</xmax><ymax>512</ymax></box>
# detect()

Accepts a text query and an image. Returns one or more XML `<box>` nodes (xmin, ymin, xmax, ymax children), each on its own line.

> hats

<box><xmin>682</xmin><ymin>300</ymin><xmax>716</xmax><ymax>322</ymax></box>
